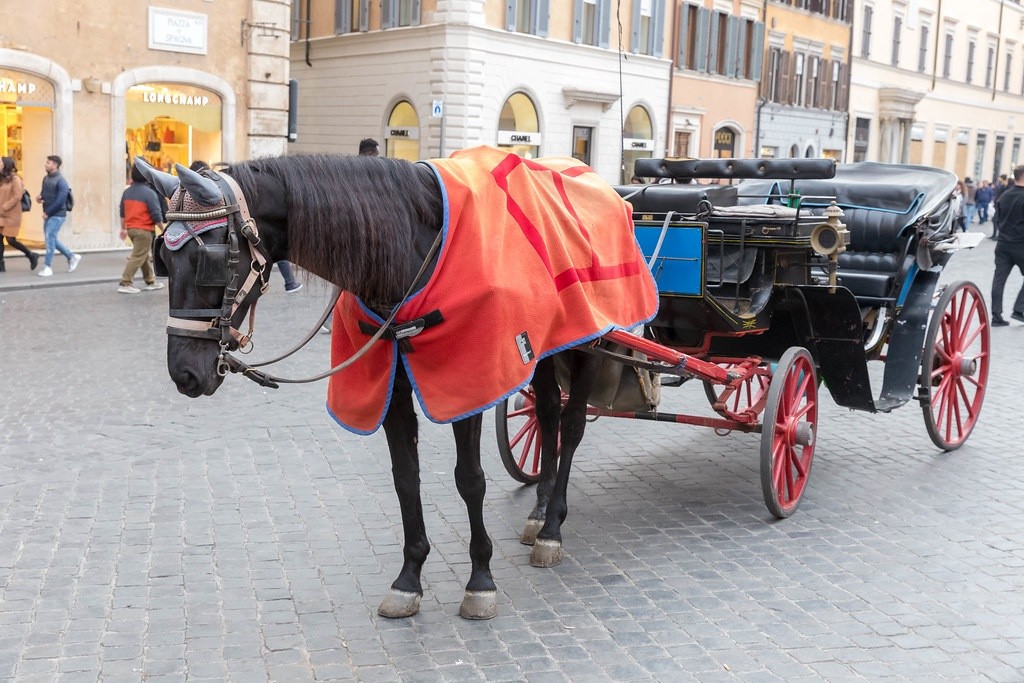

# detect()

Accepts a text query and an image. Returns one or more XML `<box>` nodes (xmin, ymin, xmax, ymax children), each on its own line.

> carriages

<box><xmin>133</xmin><ymin>144</ymin><xmax>991</xmax><ymax>619</ymax></box>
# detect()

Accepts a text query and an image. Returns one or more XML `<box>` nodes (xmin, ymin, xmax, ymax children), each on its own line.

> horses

<box><xmin>133</xmin><ymin>153</ymin><xmax>596</xmax><ymax>620</ymax></box>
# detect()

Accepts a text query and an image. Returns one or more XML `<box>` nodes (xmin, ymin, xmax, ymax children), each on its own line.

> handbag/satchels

<box><xmin>66</xmin><ymin>188</ymin><xmax>74</xmax><ymax>211</ymax></box>
<box><xmin>21</xmin><ymin>191</ymin><xmax>32</xmax><ymax>211</ymax></box>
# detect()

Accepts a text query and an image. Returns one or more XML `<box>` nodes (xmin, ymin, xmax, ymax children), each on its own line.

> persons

<box><xmin>190</xmin><ymin>159</ymin><xmax>209</xmax><ymax>173</ymax></box>
<box><xmin>277</xmin><ymin>260</ymin><xmax>302</xmax><ymax>292</ymax></box>
<box><xmin>991</xmin><ymin>165</ymin><xmax>1024</xmax><ymax>326</ymax></box>
<box><xmin>116</xmin><ymin>165</ymin><xmax>169</xmax><ymax>292</ymax></box>
<box><xmin>36</xmin><ymin>156</ymin><xmax>81</xmax><ymax>276</ymax></box>
<box><xmin>0</xmin><ymin>157</ymin><xmax>39</xmax><ymax>271</ymax></box>
<box><xmin>358</xmin><ymin>139</ymin><xmax>380</xmax><ymax>156</ymax></box>
<box><xmin>954</xmin><ymin>174</ymin><xmax>1014</xmax><ymax>241</ymax></box>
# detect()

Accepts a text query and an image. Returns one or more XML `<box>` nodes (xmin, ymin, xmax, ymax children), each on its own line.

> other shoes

<box><xmin>1011</xmin><ymin>312</ymin><xmax>1023</xmax><ymax>321</ymax></box>
<box><xmin>285</xmin><ymin>282</ymin><xmax>303</xmax><ymax>293</ymax></box>
<box><xmin>992</xmin><ymin>314</ymin><xmax>1010</xmax><ymax>326</ymax></box>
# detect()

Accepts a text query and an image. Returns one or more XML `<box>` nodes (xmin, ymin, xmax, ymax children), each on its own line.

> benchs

<box><xmin>613</xmin><ymin>156</ymin><xmax>836</xmax><ymax>286</ymax></box>
<box><xmin>747</xmin><ymin>184</ymin><xmax>920</xmax><ymax>299</ymax></box>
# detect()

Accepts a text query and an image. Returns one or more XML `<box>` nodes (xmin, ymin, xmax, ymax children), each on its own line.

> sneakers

<box><xmin>118</xmin><ymin>284</ymin><xmax>140</xmax><ymax>293</ymax></box>
<box><xmin>0</xmin><ymin>262</ymin><xmax>5</xmax><ymax>272</ymax></box>
<box><xmin>145</xmin><ymin>282</ymin><xmax>164</xmax><ymax>290</ymax></box>
<box><xmin>38</xmin><ymin>266</ymin><xmax>53</xmax><ymax>276</ymax></box>
<box><xmin>68</xmin><ymin>254</ymin><xmax>81</xmax><ymax>272</ymax></box>
<box><xmin>29</xmin><ymin>253</ymin><xmax>40</xmax><ymax>270</ymax></box>
